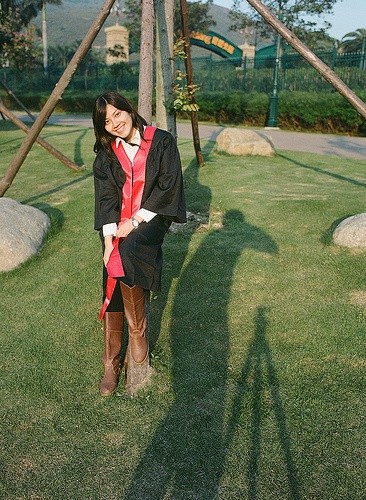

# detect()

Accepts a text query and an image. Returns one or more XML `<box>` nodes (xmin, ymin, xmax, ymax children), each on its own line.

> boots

<box><xmin>119</xmin><ymin>281</ymin><xmax>150</xmax><ymax>365</ymax></box>
<box><xmin>99</xmin><ymin>312</ymin><xmax>125</xmax><ymax>396</ymax></box>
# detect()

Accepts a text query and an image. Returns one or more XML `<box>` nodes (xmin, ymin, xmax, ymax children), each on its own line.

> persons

<box><xmin>92</xmin><ymin>92</ymin><xmax>187</xmax><ymax>396</ymax></box>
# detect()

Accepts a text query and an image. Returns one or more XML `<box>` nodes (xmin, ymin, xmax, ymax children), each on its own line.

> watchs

<box><xmin>131</xmin><ymin>216</ymin><xmax>139</xmax><ymax>230</ymax></box>
